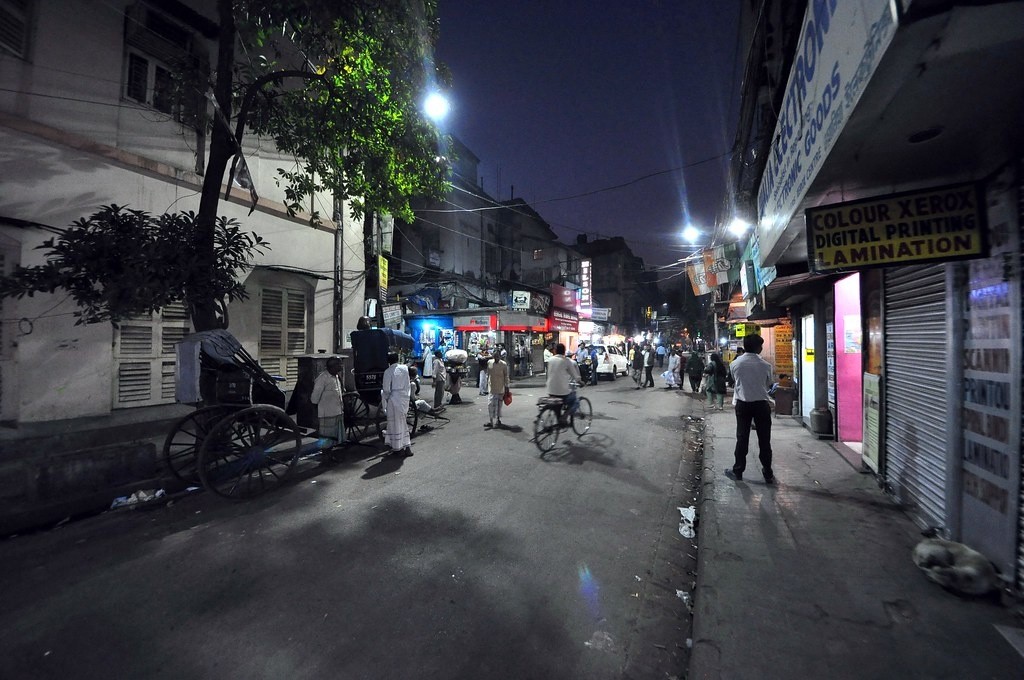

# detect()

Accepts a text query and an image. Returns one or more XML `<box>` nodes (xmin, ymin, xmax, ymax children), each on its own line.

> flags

<box><xmin>688</xmin><ymin>264</ymin><xmax>718</xmax><ymax>296</ymax></box>
<box><xmin>704</xmin><ymin>242</ymin><xmax>740</xmax><ymax>287</ymax></box>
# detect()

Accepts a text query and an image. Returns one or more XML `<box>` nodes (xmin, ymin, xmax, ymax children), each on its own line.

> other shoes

<box><xmin>332</xmin><ymin>455</ymin><xmax>343</xmax><ymax>463</ymax></box>
<box><xmin>496</xmin><ymin>420</ymin><xmax>501</xmax><ymax>425</ymax></box>
<box><xmin>484</xmin><ymin>423</ymin><xmax>493</xmax><ymax>427</ymax></box>
<box><xmin>322</xmin><ymin>459</ymin><xmax>336</xmax><ymax>466</ymax></box>
<box><xmin>765</xmin><ymin>475</ymin><xmax>775</xmax><ymax>484</ymax></box>
<box><xmin>405</xmin><ymin>448</ymin><xmax>414</xmax><ymax>456</ymax></box>
<box><xmin>388</xmin><ymin>449</ymin><xmax>404</xmax><ymax>458</ymax></box>
<box><xmin>724</xmin><ymin>469</ymin><xmax>742</xmax><ymax>480</ymax></box>
<box><xmin>420</xmin><ymin>424</ymin><xmax>434</xmax><ymax>430</ymax></box>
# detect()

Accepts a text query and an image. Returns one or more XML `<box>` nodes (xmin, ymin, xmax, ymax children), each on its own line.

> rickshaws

<box><xmin>163</xmin><ymin>329</ymin><xmax>383</xmax><ymax>500</ymax></box>
<box><xmin>332</xmin><ymin>326</ymin><xmax>451</xmax><ymax>442</ymax></box>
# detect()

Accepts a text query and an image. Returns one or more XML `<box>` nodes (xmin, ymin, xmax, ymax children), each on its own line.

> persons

<box><xmin>383</xmin><ymin>353</ymin><xmax>414</xmax><ymax>456</ymax></box>
<box><xmin>410</xmin><ymin>342</ymin><xmax>746</xmax><ymax>438</ymax></box>
<box><xmin>724</xmin><ymin>334</ymin><xmax>778</xmax><ymax>484</ymax></box>
<box><xmin>310</xmin><ymin>355</ymin><xmax>345</xmax><ymax>463</ymax></box>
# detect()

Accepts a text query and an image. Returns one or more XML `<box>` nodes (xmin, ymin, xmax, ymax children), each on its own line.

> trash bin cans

<box><xmin>810</xmin><ymin>408</ymin><xmax>833</xmax><ymax>434</ymax></box>
<box><xmin>773</xmin><ymin>385</ymin><xmax>797</xmax><ymax>415</ymax></box>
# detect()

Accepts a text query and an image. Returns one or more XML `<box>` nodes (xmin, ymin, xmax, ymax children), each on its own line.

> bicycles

<box><xmin>528</xmin><ymin>382</ymin><xmax>594</xmax><ymax>452</ymax></box>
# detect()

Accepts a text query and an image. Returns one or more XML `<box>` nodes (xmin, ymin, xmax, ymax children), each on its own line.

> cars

<box><xmin>583</xmin><ymin>344</ymin><xmax>629</xmax><ymax>380</ymax></box>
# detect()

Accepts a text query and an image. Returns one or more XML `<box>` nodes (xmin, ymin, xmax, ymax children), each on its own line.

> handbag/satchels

<box><xmin>661</xmin><ymin>371</ymin><xmax>669</xmax><ymax>378</ymax></box>
<box><xmin>503</xmin><ymin>392</ymin><xmax>512</xmax><ymax>406</ymax></box>
<box><xmin>666</xmin><ymin>373</ymin><xmax>675</xmax><ymax>385</ymax></box>
